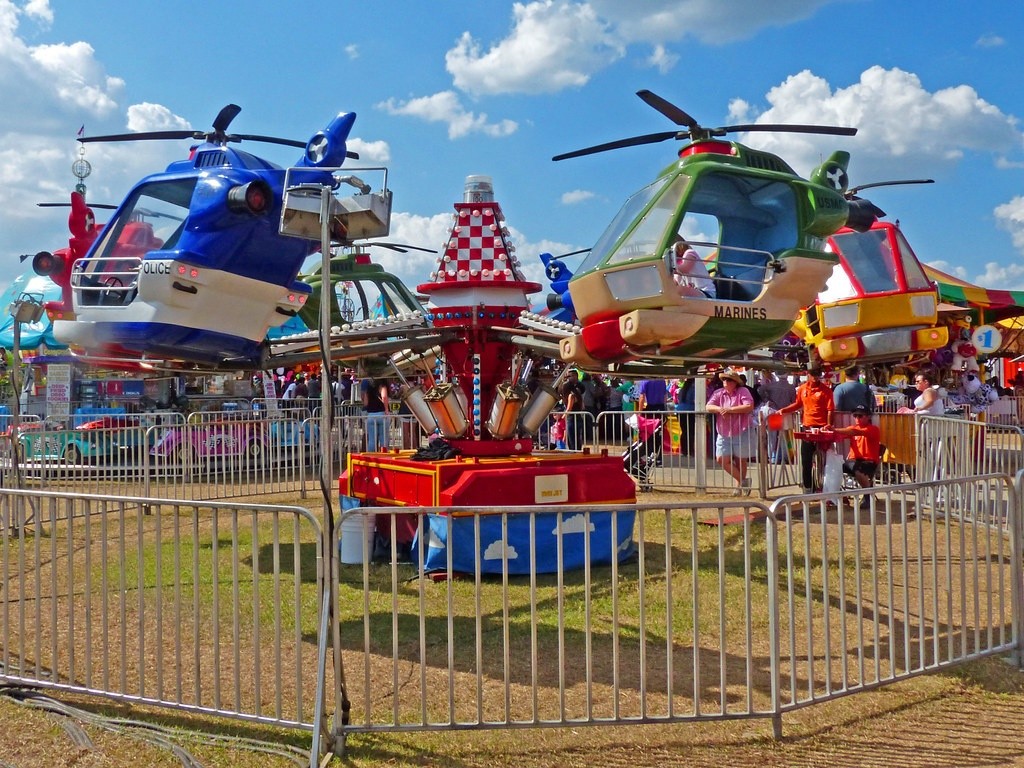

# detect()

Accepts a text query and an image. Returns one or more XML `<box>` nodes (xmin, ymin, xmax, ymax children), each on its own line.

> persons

<box><xmin>283</xmin><ymin>369</ymin><xmax>353</xmax><ymax>415</ymax></box>
<box><xmin>0</xmin><ymin>347</ymin><xmax>8</xmax><ymax>373</ymax></box>
<box><xmin>360</xmin><ymin>372</ymin><xmax>389</xmax><ymax>454</ymax></box>
<box><xmin>818</xmin><ymin>403</ymin><xmax>881</xmax><ymax>511</ymax></box>
<box><xmin>912</xmin><ymin>368</ymin><xmax>945</xmax><ymax>482</ymax></box>
<box><xmin>670</xmin><ymin>239</ymin><xmax>717</xmax><ymax>300</ymax></box>
<box><xmin>533</xmin><ymin>365</ymin><xmax>908</xmax><ymax>497</ymax></box>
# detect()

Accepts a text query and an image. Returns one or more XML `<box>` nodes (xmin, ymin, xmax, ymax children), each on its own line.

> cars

<box><xmin>0</xmin><ymin>405</ymin><xmax>320</xmax><ymax>466</ymax></box>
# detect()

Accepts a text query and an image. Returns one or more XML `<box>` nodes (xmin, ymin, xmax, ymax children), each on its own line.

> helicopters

<box><xmin>552</xmin><ymin>89</ymin><xmax>887</xmax><ymax>381</ymax></box>
<box><xmin>289</xmin><ymin>239</ymin><xmax>440</xmax><ymax>374</ymax></box>
<box><xmin>793</xmin><ymin>180</ymin><xmax>949</xmax><ymax>364</ymax></box>
<box><xmin>537</xmin><ymin>238</ymin><xmax>719</xmax><ymax>324</ymax></box>
<box><xmin>54</xmin><ymin>104</ymin><xmax>359</xmax><ymax>371</ymax></box>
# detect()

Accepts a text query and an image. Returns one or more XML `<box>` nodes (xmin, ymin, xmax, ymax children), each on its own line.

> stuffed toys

<box><xmin>949</xmin><ymin>340</ymin><xmax>999</xmax><ymax>415</ymax></box>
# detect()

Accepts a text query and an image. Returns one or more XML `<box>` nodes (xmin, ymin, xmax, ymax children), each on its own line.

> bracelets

<box><xmin>917</xmin><ymin>407</ymin><xmax>918</xmax><ymax>411</ymax></box>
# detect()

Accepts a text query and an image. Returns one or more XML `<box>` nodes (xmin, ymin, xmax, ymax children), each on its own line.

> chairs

<box><xmin>840</xmin><ymin>444</ymin><xmax>887</xmax><ymax>500</ymax></box>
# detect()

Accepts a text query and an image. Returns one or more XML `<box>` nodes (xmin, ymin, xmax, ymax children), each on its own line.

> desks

<box><xmin>793</xmin><ymin>431</ymin><xmax>848</xmax><ymax>493</ymax></box>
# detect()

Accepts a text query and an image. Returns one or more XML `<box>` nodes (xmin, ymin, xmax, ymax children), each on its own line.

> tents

<box><xmin>919</xmin><ymin>264</ymin><xmax>1024</xmax><ymax>392</ymax></box>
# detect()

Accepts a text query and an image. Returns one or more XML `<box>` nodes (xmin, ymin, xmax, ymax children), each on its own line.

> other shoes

<box><xmin>740</xmin><ymin>478</ymin><xmax>752</xmax><ymax>496</ymax></box>
<box><xmin>732</xmin><ymin>484</ymin><xmax>742</xmax><ymax>496</ymax></box>
<box><xmin>860</xmin><ymin>495</ymin><xmax>870</xmax><ymax>509</ymax></box>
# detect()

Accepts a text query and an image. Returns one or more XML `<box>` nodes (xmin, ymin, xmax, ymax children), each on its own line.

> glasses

<box><xmin>853</xmin><ymin>414</ymin><xmax>863</xmax><ymax>418</ymax></box>
<box><xmin>722</xmin><ymin>377</ymin><xmax>732</xmax><ymax>382</ymax></box>
<box><xmin>810</xmin><ymin>372</ymin><xmax>821</xmax><ymax>376</ymax></box>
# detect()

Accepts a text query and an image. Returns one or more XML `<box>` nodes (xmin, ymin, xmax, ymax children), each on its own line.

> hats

<box><xmin>718</xmin><ymin>370</ymin><xmax>745</xmax><ymax>386</ymax></box>
<box><xmin>806</xmin><ymin>361</ymin><xmax>822</xmax><ymax>376</ymax></box>
<box><xmin>851</xmin><ymin>404</ymin><xmax>873</xmax><ymax>415</ymax></box>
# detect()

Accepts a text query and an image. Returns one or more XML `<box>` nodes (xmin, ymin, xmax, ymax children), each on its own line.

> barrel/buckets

<box><xmin>341</xmin><ymin>511</ymin><xmax>376</xmax><ymax>564</ymax></box>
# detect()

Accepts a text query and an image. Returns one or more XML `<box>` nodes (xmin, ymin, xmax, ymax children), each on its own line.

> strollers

<box><xmin>620</xmin><ymin>409</ymin><xmax>668</xmax><ymax>493</ymax></box>
